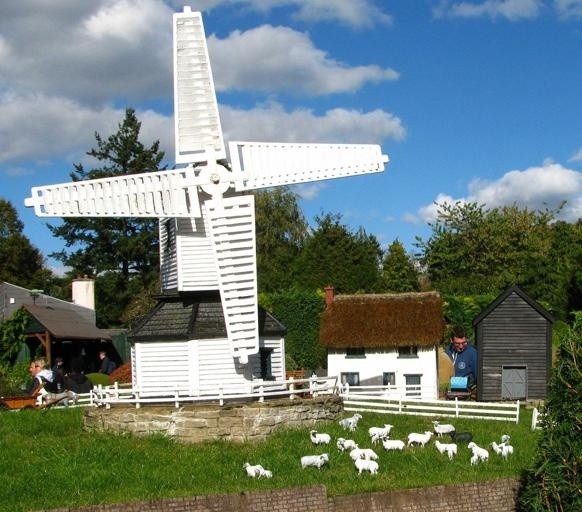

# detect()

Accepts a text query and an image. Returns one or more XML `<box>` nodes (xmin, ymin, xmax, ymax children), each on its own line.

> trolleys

<box><xmin>0</xmin><ymin>382</ymin><xmax>70</xmax><ymax>412</ymax></box>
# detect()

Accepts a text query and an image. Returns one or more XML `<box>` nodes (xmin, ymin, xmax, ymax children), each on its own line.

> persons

<box><xmin>26</xmin><ymin>357</ymin><xmax>93</xmax><ymax>396</ymax></box>
<box><xmin>98</xmin><ymin>351</ymin><xmax>115</xmax><ymax>375</ymax></box>
<box><xmin>446</xmin><ymin>328</ymin><xmax>477</xmax><ymax>393</ymax></box>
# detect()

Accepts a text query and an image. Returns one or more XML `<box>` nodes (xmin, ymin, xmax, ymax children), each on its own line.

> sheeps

<box><xmin>336</xmin><ymin>438</ymin><xmax>356</xmax><ymax>453</ymax></box>
<box><xmin>449</xmin><ymin>431</ymin><xmax>473</xmax><ymax>443</ymax></box>
<box><xmin>407</xmin><ymin>430</ymin><xmax>434</xmax><ymax>448</ymax></box>
<box><xmin>368</xmin><ymin>424</ymin><xmax>405</xmax><ymax>452</ymax></box>
<box><xmin>350</xmin><ymin>444</ymin><xmax>379</xmax><ymax>475</ymax></box>
<box><xmin>431</xmin><ymin>421</ymin><xmax>456</xmax><ymax>437</ymax></box>
<box><xmin>467</xmin><ymin>442</ymin><xmax>489</xmax><ymax>466</ymax></box>
<box><xmin>301</xmin><ymin>453</ymin><xmax>329</xmax><ymax>473</ymax></box>
<box><xmin>243</xmin><ymin>462</ymin><xmax>273</xmax><ymax>480</ymax></box>
<box><xmin>309</xmin><ymin>429</ymin><xmax>331</xmax><ymax>447</ymax></box>
<box><xmin>489</xmin><ymin>435</ymin><xmax>514</xmax><ymax>462</ymax></box>
<box><xmin>338</xmin><ymin>413</ymin><xmax>363</xmax><ymax>432</ymax></box>
<box><xmin>434</xmin><ymin>440</ymin><xmax>457</xmax><ymax>460</ymax></box>
